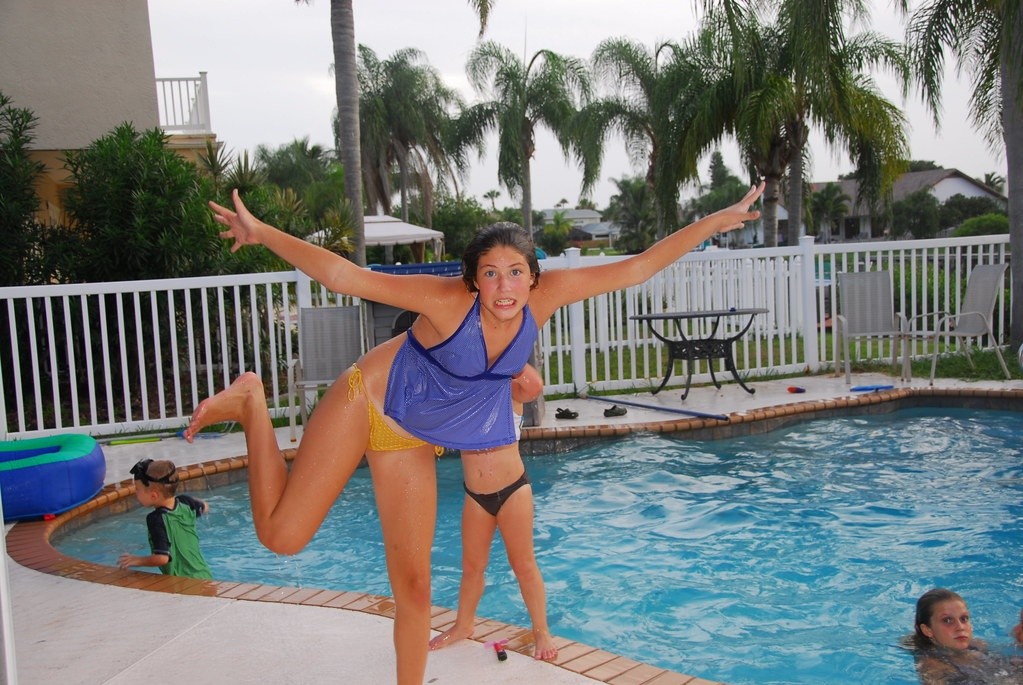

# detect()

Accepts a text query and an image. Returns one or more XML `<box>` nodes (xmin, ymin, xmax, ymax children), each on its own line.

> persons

<box><xmin>429</xmin><ymin>362</ymin><xmax>559</xmax><ymax>659</ymax></box>
<box><xmin>116</xmin><ymin>459</ymin><xmax>213</xmax><ymax>579</ymax></box>
<box><xmin>183</xmin><ymin>181</ymin><xmax>765</xmax><ymax>685</ymax></box>
<box><xmin>904</xmin><ymin>588</ymin><xmax>972</xmax><ymax>652</ymax></box>
<box><xmin>1012</xmin><ymin>612</ymin><xmax>1023</xmax><ymax>648</ymax></box>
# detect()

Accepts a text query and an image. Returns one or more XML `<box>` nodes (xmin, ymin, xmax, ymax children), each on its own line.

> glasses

<box><xmin>130</xmin><ymin>459</ymin><xmax>153</xmax><ymax>482</ymax></box>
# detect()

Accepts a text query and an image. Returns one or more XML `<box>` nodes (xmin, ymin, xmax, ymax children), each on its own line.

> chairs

<box><xmin>905</xmin><ymin>263</ymin><xmax>1016</xmax><ymax>385</ymax></box>
<box><xmin>834</xmin><ymin>269</ymin><xmax>909</xmax><ymax>385</ymax></box>
<box><xmin>285</xmin><ymin>306</ymin><xmax>366</xmax><ymax>441</ymax></box>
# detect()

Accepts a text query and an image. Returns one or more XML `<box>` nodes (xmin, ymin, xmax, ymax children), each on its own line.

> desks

<box><xmin>629</xmin><ymin>308</ymin><xmax>769</xmax><ymax>400</ymax></box>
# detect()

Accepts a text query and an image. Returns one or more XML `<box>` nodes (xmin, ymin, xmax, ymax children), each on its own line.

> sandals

<box><xmin>603</xmin><ymin>407</ymin><xmax>626</xmax><ymax>417</ymax></box>
<box><xmin>554</xmin><ymin>408</ymin><xmax>579</xmax><ymax>419</ymax></box>
